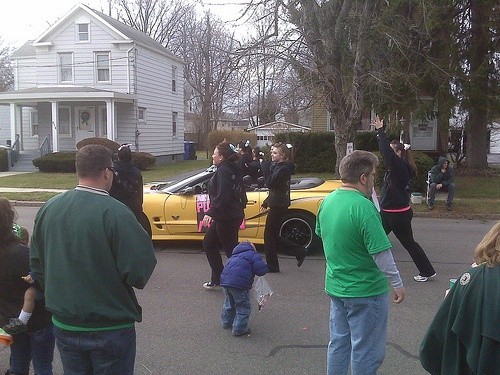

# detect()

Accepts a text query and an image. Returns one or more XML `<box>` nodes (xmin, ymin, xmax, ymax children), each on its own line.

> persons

<box><xmin>202</xmin><ymin>142</ymin><xmax>248</xmax><ymax>288</ymax></box>
<box><xmin>419</xmin><ymin>223</ymin><xmax>500</xmax><ymax>375</ymax></box>
<box><xmin>3</xmin><ymin>223</ymin><xmax>43</xmax><ymax>335</ymax></box>
<box><xmin>0</xmin><ymin>197</ymin><xmax>55</xmax><ymax>375</ymax></box>
<box><xmin>428</xmin><ymin>158</ymin><xmax>456</xmax><ymax>211</ymax></box>
<box><xmin>370</xmin><ymin>115</ymin><xmax>436</xmax><ymax>282</ymax></box>
<box><xmin>315</xmin><ymin>150</ymin><xmax>406</xmax><ymax>375</ymax></box>
<box><xmin>29</xmin><ymin>143</ymin><xmax>157</xmax><ymax>375</ymax></box>
<box><xmin>260</xmin><ymin>142</ymin><xmax>307</xmax><ymax>273</ymax></box>
<box><xmin>229</xmin><ymin>143</ymin><xmax>265</xmax><ymax>192</ymax></box>
<box><xmin>219</xmin><ymin>240</ymin><xmax>269</xmax><ymax>336</ymax></box>
<box><xmin>111</xmin><ymin>144</ymin><xmax>143</xmax><ymax>225</ymax></box>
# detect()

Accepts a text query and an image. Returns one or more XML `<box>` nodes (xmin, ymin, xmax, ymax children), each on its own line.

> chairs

<box><xmin>426</xmin><ymin>170</ymin><xmax>448</xmax><ymax>204</ymax></box>
<box><xmin>243</xmin><ymin>174</ymin><xmax>252</xmax><ymax>187</ymax></box>
<box><xmin>258</xmin><ymin>176</ymin><xmax>265</xmax><ymax>189</ymax></box>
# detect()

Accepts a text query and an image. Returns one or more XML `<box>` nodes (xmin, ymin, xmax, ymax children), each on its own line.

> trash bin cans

<box><xmin>184</xmin><ymin>141</ymin><xmax>197</xmax><ymax>160</ymax></box>
<box><xmin>0</xmin><ymin>145</ymin><xmax>12</xmax><ymax>172</ymax></box>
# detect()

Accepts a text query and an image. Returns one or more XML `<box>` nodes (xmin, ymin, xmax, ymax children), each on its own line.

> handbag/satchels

<box><xmin>251</xmin><ymin>276</ymin><xmax>274</xmax><ymax>313</ymax></box>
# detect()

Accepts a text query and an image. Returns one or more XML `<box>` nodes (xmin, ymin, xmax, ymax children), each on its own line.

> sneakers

<box><xmin>413</xmin><ymin>273</ymin><xmax>436</xmax><ymax>282</ymax></box>
<box><xmin>3</xmin><ymin>317</ymin><xmax>28</xmax><ymax>334</ymax></box>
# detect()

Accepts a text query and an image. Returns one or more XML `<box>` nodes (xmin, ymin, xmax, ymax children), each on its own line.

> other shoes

<box><xmin>447</xmin><ymin>202</ymin><xmax>452</xmax><ymax>211</ymax></box>
<box><xmin>266</xmin><ymin>265</ymin><xmax>280</xmax><ymax>272</ymax></box>
<box><xmin>428</xmin><ymin>200</ymin><xmax>433</xmax><ymax>209</ymax></box>
<box><xmin>235</xmin><ymin>328</ymin><xmax>251</xmax><ymax>337</ymax></box>
<box><xmin>297</xmin><ymin>247</ymin><xmax>307</xmax><ymax>267</ymax></box>
<box><xmin>202</xmin><ymin>282</ymin><xmax>223</xmax><ymax>289</ymax></box>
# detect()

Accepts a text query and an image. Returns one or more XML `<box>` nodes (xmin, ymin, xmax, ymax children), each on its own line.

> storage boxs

<box><xmin>411</xmin><ymin>192</ymin><xmax>422</xmax><ymax>204</ymax></box>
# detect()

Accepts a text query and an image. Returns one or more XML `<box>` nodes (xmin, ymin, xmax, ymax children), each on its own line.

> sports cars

<box><xmin>142</xmin><ymin>164</ymin><xmax>343</xmax><ymax>256</ymax></box>
